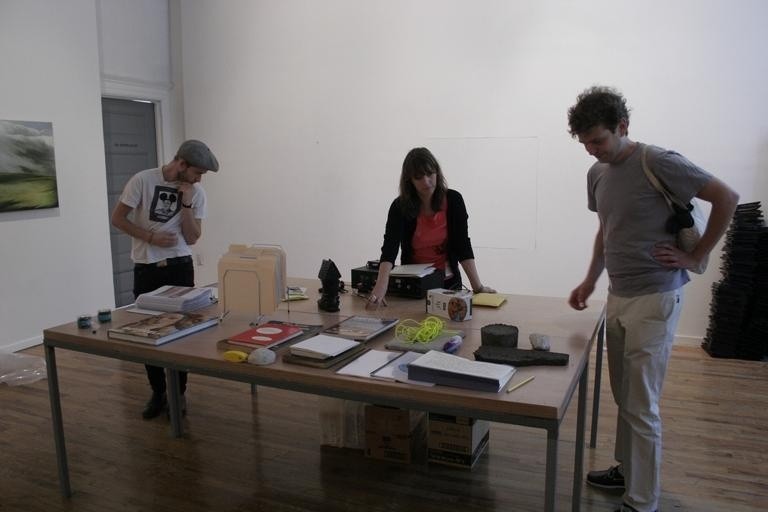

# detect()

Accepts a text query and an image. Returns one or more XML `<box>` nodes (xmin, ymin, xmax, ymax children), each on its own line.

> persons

<box><xmin>566</xmin><ymin>85</ymin><xmax>739</xmax><ymax>511</ymax></box>
<box><xmin>109</xmin><ymin>139</ymin><xmax>221</xmax><ymax>425</ymax></box>
<box><xmin>363</xmin><ymin>147</ymin><xmax>497</xmax><ymax>310</ymax></box>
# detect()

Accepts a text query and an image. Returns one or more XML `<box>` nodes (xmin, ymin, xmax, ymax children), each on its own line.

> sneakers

<box><xmin>142</xmin><ymin>391</ymin><xmax>187</xmax><ymax>420</ymax></box>
<box><xmin>587</xmin><ymin>465</ymin><xmax>626</xmax><ymax>490</ymax></box>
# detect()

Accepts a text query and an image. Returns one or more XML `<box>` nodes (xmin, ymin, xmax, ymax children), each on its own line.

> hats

<box><xmin>176</xmin><ymin>140</ymin><xmax>219</xmax><ymax>172</ymax></box>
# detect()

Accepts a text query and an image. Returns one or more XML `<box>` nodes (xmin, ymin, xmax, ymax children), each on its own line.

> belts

<box><xmin>134</xmin><ymin>255</ymin><xmax>192</xmax><ymax>269</ymax></box>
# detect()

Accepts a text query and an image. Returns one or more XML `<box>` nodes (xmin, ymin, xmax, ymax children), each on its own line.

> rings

<box><xmin>368</xmin><ymin>293</ymin><xmax>377</xmax><ymax>302</ymax></box>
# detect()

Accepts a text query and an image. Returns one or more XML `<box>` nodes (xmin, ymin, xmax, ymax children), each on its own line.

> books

<box><xmin>281</xmin><ymin>315</ymin><xmax>399</xmax><ymax>370</ymax></box>
<box><xmin>107</xmin><ymin>309</ymin><xmax>219</xmax><ymax>347</ymax></box>
<box><xmin>385</xmin><ymin>328</ymin><xmax>465</xmax><ymax>355</ymax></box>
<box><xmin>333</xmin><ymin>346</ymin><xmax>435</xmax><ymax>387</ymax></box>
<box><xmin>216</xmin><ymin>318</ymin><xmax>325</xmax><ymax>352</ymax></box>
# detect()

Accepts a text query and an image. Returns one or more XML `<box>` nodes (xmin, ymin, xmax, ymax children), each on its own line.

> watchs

<box><xmin>180</xmin><ymin>201</ymin><xmax>194</xmax><ymax>209</ymax></box>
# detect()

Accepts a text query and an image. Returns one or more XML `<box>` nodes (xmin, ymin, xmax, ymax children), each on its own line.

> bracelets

<box><xmin>147</xmin><ymin>231</ymin><xmax>155</xmax><ymax>244</ymax></box>
<box><xmin>473</xmin><ymin>285</ymin><xmax>484</xmax><ymax>294</ymax></box>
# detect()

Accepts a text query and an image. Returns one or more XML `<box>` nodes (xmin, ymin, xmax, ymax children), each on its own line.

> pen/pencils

<box><xmin>507</xmin><ymin>376</ymin><xmax>535</xmax><ymax>393</ymax></box>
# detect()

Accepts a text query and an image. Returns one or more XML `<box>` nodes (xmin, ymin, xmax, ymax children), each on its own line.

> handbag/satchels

<box><xmin>640</xmin><ymin>143</ymin><xmax>708</xmax><ymax>274</ymax></box>
